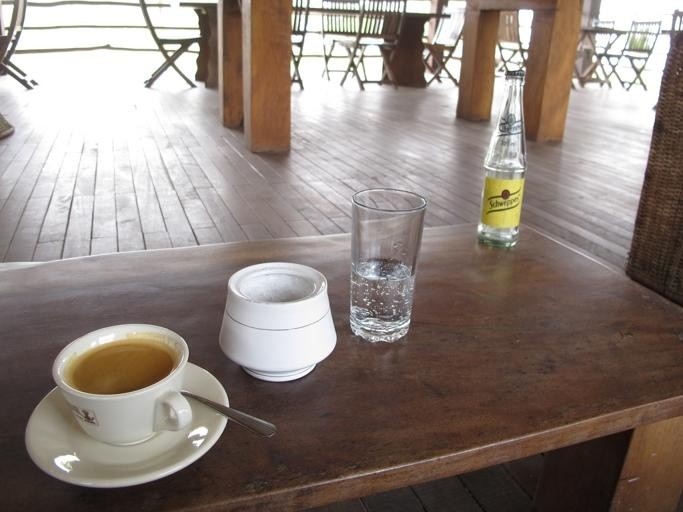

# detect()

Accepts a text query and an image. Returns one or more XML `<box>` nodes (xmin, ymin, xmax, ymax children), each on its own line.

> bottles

<box><xmin>476</xmin><ymin>70</ymin><xmax>528</xmax><ymax>247</ymax></box>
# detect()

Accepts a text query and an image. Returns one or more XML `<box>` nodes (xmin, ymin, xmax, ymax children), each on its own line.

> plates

<box><xmin>22</xmin><ymin>360</ymin><xmax>231</xmax><ymax>489</ymax></box>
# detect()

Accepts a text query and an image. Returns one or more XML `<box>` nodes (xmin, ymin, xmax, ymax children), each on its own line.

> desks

<box><xmin>0</xmin><ymin>221</ymin><xmax>683</xmax><ymax>511</ymax></box>
<box><xmin>179</xmin><ymin>2</ymin><xmax>217</xmax><ymax>89</ymax></box>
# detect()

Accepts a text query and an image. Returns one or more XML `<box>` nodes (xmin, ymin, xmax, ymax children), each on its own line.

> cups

<box><xmin>52</xmin><ymin>323</ymin><xmax>192</xmax><ymax>448</ymax></box>
<box><xmin>350</xmin><ymin>187</ymin><xmax>427</xmax><ymax>344</ymax></box>
<box><xmin>218</xmin><ymin>262</ymin><xmax>338</xmax><ymax>382</ymax></box>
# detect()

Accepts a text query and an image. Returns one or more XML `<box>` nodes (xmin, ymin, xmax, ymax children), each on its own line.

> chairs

<box><xmin>495</xmin><ymin>8</ymin><xmax>662</xmax><ymax>92</ymax></box>
<box><xmin>290</xmin><ymin>0</ymin><xmax>466</xmax><ymax>90</ymax></box>
<box><xmin>1</xmin><ymin>1</ymin><xmax>38</xmax><ymax>90</ymax></box>
<box><xmin>139</xmin><ymin>0</ymin><xmax>206</xmax><ymax>88</ymax></box>
